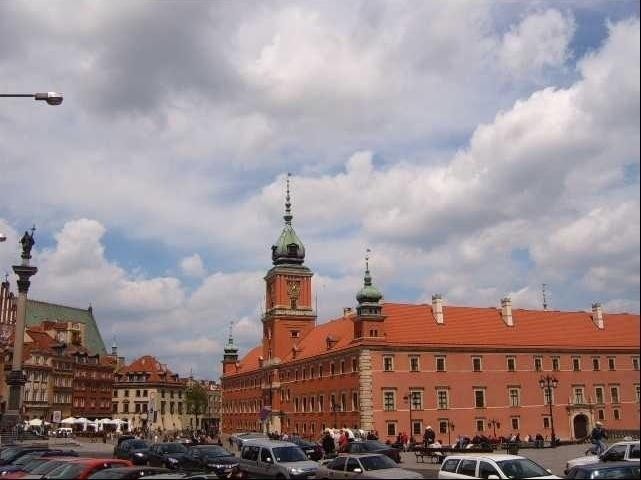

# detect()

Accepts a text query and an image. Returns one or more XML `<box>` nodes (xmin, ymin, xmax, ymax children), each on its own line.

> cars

<box><xmin>284</xmin><ymin>437</ymin><xmax>323</xmax><ymax>462</ymax></box>
<box><xmin>138</xmin><ymin>468</ymin><xmax>219</xmax><ymax>480</ymax></box>
<box><xmin>146</xmin><ymin>442</ymin><xmax>190</xmax><ymax>470</ymax></box>
<box><xmin>180</xmin><ymin>444</ymin><xmax>240</xmax><ymax>480</ymax></box>
<box><xmin>327</xmin><ymin>452</ymin><xmax>424</xmax><ymax>480</ymax></box>
<box><xmin>567</xmin><ymin>459</ymin><xmax>641</xmax><ymax>480</ymax></box>
<box><xmin>330</xmin><ymin>439</ymin><xmax>402</xmax><ymax>465</ymax></box>
<box><xmin>117</xmin><ymin>435</ymin><xmax>134</xmax><ymax>445</ymax></box>
<box><xmin>1</xmin><ymin>441</ymin><xmax>132</xmax><ymax>480</ymax></box>
<box><xmin>114</xmin><ymin>439</ymin><xmax>152</xmax><ymax>466</ymax></box>
<box><xmin>88</xmin><ymin>464</ymin><xmax>174</xmax><ymax>480</ymax></box>
<box><xmin>438</xmin><ymin>453</ymin><xmax>566</xmax><ymax>480</ymax></box>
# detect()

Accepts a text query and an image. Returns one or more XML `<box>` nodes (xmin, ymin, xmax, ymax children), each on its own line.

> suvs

<box><xmin>237</xmin><ymin>439</ymin><xmax>322</xmax><ymax>480</ymax></box>
<box><xmin>563</xmin><ymin>439</ymin><xmax>640</xmax><ymax>475</ymax></box>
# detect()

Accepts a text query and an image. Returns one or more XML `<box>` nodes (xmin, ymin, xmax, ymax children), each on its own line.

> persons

<box><xmin>423</xmin><ymin>427</ymin><xmax>563</xmax><ymax>455</ymax></box>
<box><xmin>320</xmin><ymin>431</ymin><xmax>336</xmax><ymax>455</ymax></box>
<box><xmin>336</xmin><ymin>430</ymin><xmax>350</xmax><ymax>451</ymax></box>
<box><xmin>268</xmin><ymin>430</ymin><xmax>290</xmax><ymax>441</ymax></box>
<box><xmin>354</xmin><ymin>431</ymin><xmax>410</xmax><ymax>455</ymax></box>
<box><xmin>585</xmin><ymin>421</ymin><xmax>609</xmax><ymax>457</ymax></box>
<box><xmin>102</xmin><ymin>426</ymin><xmax>224</xmax><ymax>445</ymax></box>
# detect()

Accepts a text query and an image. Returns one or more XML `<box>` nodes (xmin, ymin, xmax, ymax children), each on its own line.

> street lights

<box><xmin>0</xmin><ymin>232</ymin><xmax>7</xmax><ymax>244</ymax></box>
<box><xmin>0</xmin><ymin>90</ymin><xmax>66</xmax><ymax>108</ymax></box>
<box><xmin>537</xmin><ymin>374</ymin><xmax>559</xmax><ymax>447</ymax></box>
<box><xmin>329</xmin><ymin>397</ymin><xmax>343</xmax><ymax>434</ymax></box>
<box><xmin>403</xmin><ymin>385</ymin><xmax>421</xmax><ymax>446</ymax></box>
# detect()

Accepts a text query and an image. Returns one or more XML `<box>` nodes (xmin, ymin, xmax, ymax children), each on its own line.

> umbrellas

<box><xmin>26</xmin><ymin>416</ymin><xmax>129</xmax><ymax>433</ymax></box>
